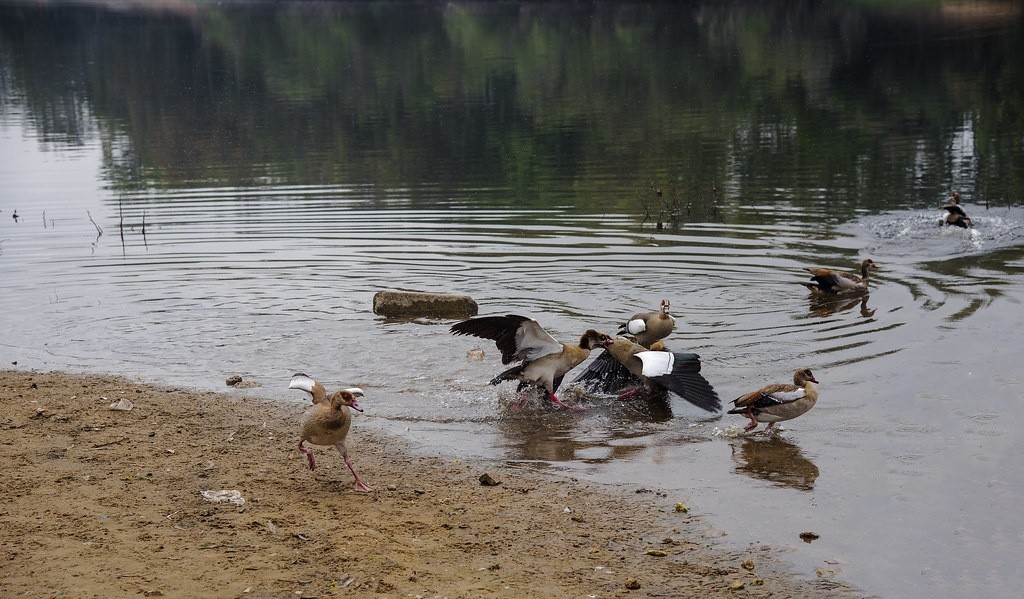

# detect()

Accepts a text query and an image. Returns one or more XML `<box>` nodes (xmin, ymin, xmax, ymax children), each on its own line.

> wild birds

<box><xmin>799</xmin><ymin>258</ymin><xmax>880</xmax><ymax>296</ymax></box>
<box><xmin>569</xmin><ymin>329</ymin><xmax>724</xmax><ymax>415</ymax></box>
<box><xmin>727</xmin><ymin>368</ymin><xmax>819</xmax><ymax>433</ymax></box>
<box><xmin>941</xmin><ymin>192</ymin><xmax>973</xmax><ymax>229</ymax></box>
<box><xmin>288</xmin><ymin>371</ymin><xmax>376</xmax><ymax>493</ymax></box>
<box><xmin>615</xmin><ymin>298</ymin><xmax>678</xmax><ymax>350</ymax></box>
<box><xmin>449</xmin><ymin>313</ymin><xmax>614</xmax><ymax>410</ymax></box>
